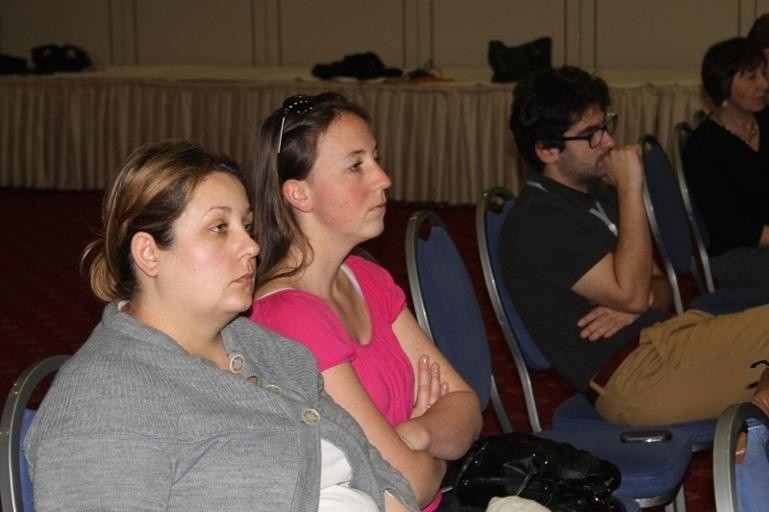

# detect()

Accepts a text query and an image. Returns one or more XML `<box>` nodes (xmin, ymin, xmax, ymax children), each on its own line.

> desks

<box><xmin>0</xmin><ymin>60</ymin><xmax>714</xmax><ymax>207</ymax></box>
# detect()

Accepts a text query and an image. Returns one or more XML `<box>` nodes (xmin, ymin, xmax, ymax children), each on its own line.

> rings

<box><xmin>427</xmin><ymin>404</ymin><xmax>431</xmax><ymax>408</ymax></box>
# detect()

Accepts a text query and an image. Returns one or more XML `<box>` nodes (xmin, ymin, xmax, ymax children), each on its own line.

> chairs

<box><xmin>405</xmin><ymin>207</ymin><xmax>694</xmax><ymax>511</ymax></box>
<box><xmin>670</xmin><ymin>122</ymin><xmax>721</xmax><ymax>293</ymax></box>
<box><xmin>0</xmin><ymin>353</ymin><xmax>71</xmax><ymax>512</ymax></box>
<box><xmin>637</xmin><ymin>131</ymin><xmax>709</xmax><ymax>318</ymax></box>
<box><xmin>477</xmin><ymin>184</ymin><xmax>721</xmax><ymax>453</ymax></box>
<box><xmin>711</xmin><ymin>399</ymin><xmax>768</xmax><ymax>512</ymax></box>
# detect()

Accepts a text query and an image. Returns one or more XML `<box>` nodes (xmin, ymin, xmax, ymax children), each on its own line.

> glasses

<box><xmin>276</xmin><ymin>92</ymin><xmax>349</xmax><ymax>160</ymax></box>
<box><xmin>556</xmin><ymin>111</ymin><xmax>618</xmax><ymax>150</ymax></box>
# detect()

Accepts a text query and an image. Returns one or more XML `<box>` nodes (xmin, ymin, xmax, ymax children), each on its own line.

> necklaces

<box><xmin>716</xmin><ymin>113</ymin><xmax>758</xmax><ymax>146</ymax></box>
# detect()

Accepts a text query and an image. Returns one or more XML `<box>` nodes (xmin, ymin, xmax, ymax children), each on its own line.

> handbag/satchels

<box><xmin>488</xmin><ymin>35</ymin><xmax>554</xmax><ymax>84</ymax></box>
<box><xmin>443</xmin><ymin>430</ymin><xmax>627</xmax><ymax>512</ymax></box>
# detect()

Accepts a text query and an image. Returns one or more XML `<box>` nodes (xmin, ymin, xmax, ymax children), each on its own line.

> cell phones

<box><xmin>620</xmin><ymin>431</ymin><xmax>673</xmax><ymax>442</ymax></box>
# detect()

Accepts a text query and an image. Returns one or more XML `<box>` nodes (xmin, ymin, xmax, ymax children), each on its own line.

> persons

<box><xmin>686</xmin><ymin>36</ymin><xmax>768</xmax><ymax>289</ymax></box>
<box><xmin>499</xmin><ymin>61</ymin><xmax>768</xmax><ymax>425</ymax></box>
<box><xmin>22</xmin><ymin>142</ymin><xmax>422</xmax><ymax>512</ymax></box>
<box><xmin>241</xmin><ymin>92</ymin><xmax>628</xmax><ymax>509</ymax></box>
